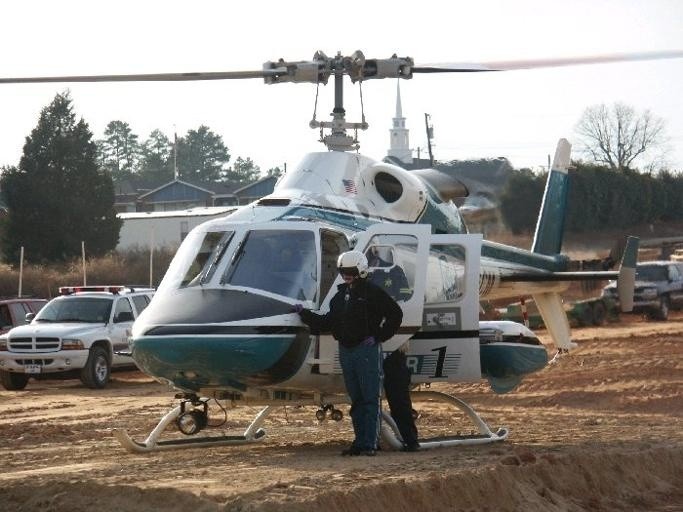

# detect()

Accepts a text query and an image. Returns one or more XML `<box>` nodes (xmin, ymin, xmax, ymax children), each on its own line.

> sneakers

<box><xmin>342</xmin><ymin>449</ymin><xmax>359</xmax><ymax>455</ymax></box>
<box><xmin>406</xmin><ymin>441</ymin><xmax>419</xmax><ymax>450</ymax></box>
<box><xmin>360</xmin><ymin>449</ymin><xmax>377</xmax><ymax>455</ymax></box>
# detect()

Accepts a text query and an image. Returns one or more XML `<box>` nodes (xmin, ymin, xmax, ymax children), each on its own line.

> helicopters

<box><xmin>0</xmin><ymin>49</ymin><xmax>683</xmax><ymax>456</ymax></box>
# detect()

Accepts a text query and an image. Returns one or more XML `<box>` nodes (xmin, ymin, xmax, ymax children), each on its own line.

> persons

<box><xmin>295</xmin><ymin>251</ymin><xmax>403</xmax><ymax>455</ymax></box>
<box><xmin>349</xmin><ymin>230</ymin><xmax>419</xmax><ymax>450</ymax></box>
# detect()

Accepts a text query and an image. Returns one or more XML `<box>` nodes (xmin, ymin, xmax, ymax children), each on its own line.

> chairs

<box><xmin>300</xmin><ymin>239</ymin><xmax>339</xmax><ymax>308</ymax></box>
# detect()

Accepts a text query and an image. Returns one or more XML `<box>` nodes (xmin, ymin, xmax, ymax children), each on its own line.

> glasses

<box><xmin>339</xmin><ymin>268</ymin><xmax>358</xmax><ymax>276</ymax></box>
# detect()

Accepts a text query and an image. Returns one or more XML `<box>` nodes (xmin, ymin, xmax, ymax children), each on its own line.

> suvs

<box><xmin>0</xmin><ymin>296</ymin><xmax>58</xmax><ymax>334</ymax></box>
<box><xmin>598</xmin><ymin>259</ymin><xmax>683</xmax><ymax>320</ymax></box>
<box><xmin>0</xmin><ymin>280</ymin><xmax>166</xmax><ymax>395</ymax></box>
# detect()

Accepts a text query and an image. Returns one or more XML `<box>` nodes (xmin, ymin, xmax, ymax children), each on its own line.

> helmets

<box><xmin>337</xmin><ymin>252</ymin><xmax>368</xmax><ymax>278</ymax></box>
<box><xmin>349</xmin><ymin>231</ymin><xmax>380</xmax><ymax>255</ymax></box>
